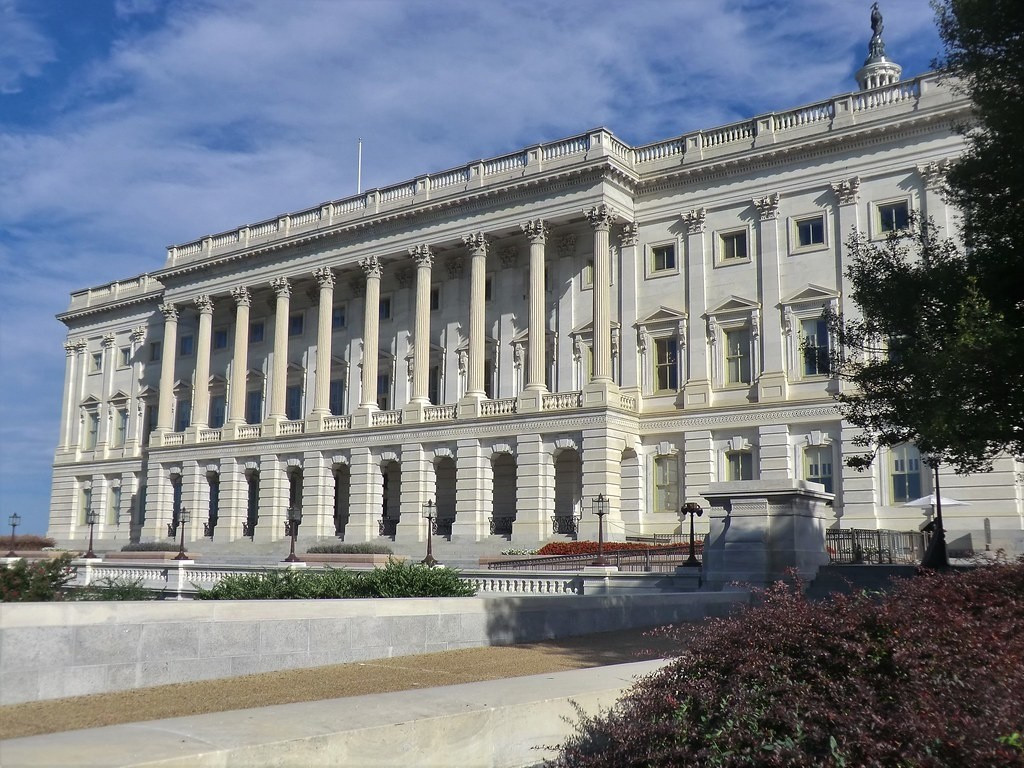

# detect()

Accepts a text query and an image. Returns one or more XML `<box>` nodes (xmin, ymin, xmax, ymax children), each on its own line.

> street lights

<box><xmin>421</xmin><ymin>499</ymin><xmax>438</xmax><ymax>563</ymax></box>
<box><xmin>681</xmin><ymin>502</ymin><xmax>703</xmax><ymax>566</ymax></box>
<box><xmin>83</xmin><ymin>510</ymin><xmax>100</xmax><ymax>558</ymax></box>
<box><xmin>174</xmin><ymin>506</ymin><xmax>191</xmax><ymax>560</ymax></box>
<box><xmin>591</xmin><ymin>493</ymin><xmax>610</xmax><ymax>566</ymax></box>
<box><xmin>285</xmin><ymin>504</ymin><xmax>300</xmax><ymax>562</ymax></box>
<box><xmin>4</xmin><ymin>512</ymin><xmax>21</xmax><ymax>557</ymax></box>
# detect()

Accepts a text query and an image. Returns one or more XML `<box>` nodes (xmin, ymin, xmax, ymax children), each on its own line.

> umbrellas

<box><xmin>896</xmin><ymin>489</ymin><xmax>968</xmax><ymax>521</ymax></box>
